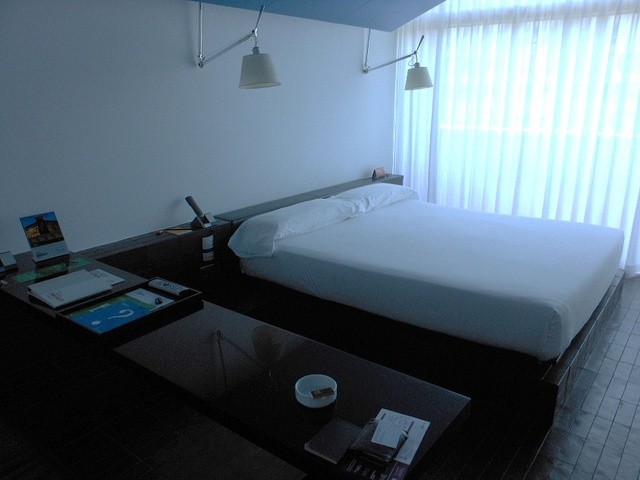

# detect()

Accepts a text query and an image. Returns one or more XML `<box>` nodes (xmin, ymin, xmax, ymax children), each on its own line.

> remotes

<box><xmin>148</xmin><ymin>277</ymin><xmax>189</xmax><ymax>296</ymax></box>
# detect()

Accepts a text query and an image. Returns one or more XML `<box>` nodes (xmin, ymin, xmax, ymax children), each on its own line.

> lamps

<box><xmin>216</xmin><ymin>324</ymin><xmax>290</xmax><ymax>392</ymax></box>
<box><xmin>198</xmin><ymin>2</ymin><xmax>281</xmax><ymax>90</ymax></box>
<box><xmin>364</xmin><ymin>27</ymin><xmax>433</xmax><ymax>90</ymax></box>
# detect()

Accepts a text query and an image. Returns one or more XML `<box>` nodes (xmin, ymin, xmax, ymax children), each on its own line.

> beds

<box><xmin>229</xmin><ymin>182</ymin><xmax>626</xmax><ymax>425</ymax></box>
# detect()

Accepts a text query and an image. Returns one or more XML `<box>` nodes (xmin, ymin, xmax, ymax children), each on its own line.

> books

<box><xmin>304</xmin><ymin>418</ymin><xmax>362</xmax><ymax>465</ymax></box>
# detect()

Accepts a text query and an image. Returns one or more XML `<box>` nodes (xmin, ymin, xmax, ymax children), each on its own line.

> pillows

<box><xmin>329</xmin><ymin>183</ymin><xmax>419</xmax><ymax>218</ymax></box>
<box><xmin>227</xmin><ymin>198</ymin><xmax>353</xmax><ymax>258</ymax></box>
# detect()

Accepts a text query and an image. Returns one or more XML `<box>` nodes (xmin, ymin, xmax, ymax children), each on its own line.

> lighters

<box><xmin>311</xmin><ymin>387</ymin><xmax>334</xmax><ymax>398</ymax></box>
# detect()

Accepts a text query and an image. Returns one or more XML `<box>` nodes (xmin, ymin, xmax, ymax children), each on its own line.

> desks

<box><xmin>0</xmin><ymin>250</ymin><xmax>473</xmax><ymax>479</ymax></box>
